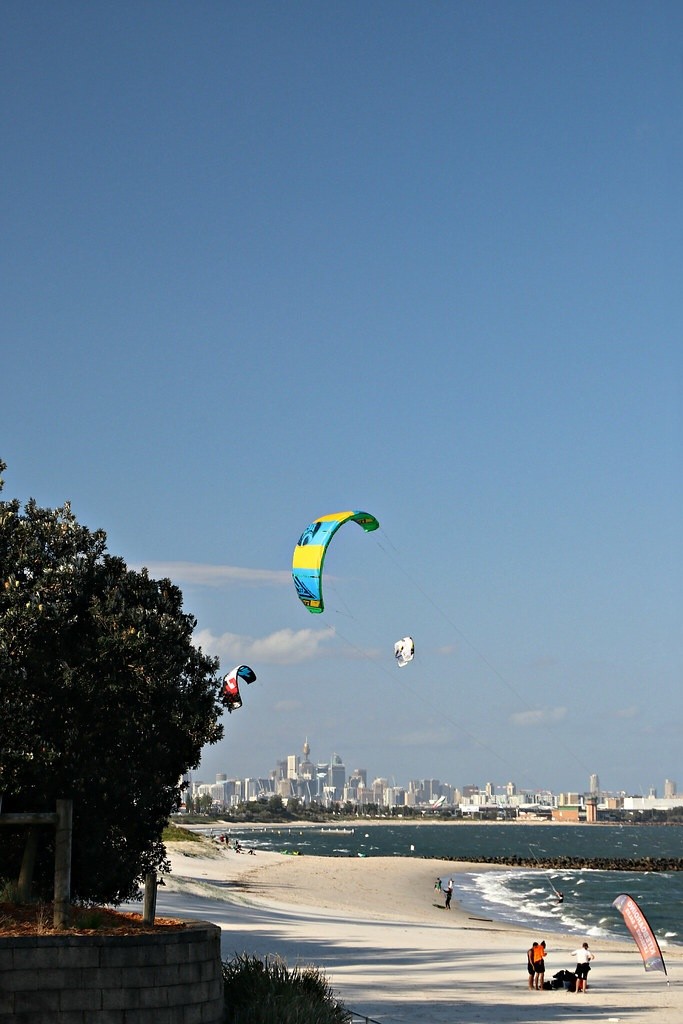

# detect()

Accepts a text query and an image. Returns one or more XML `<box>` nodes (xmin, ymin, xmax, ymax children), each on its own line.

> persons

<box><xmin>571</xmin><ymin>943</ymin><xmax>595</xmax><ymax>994</ymax></box>
<box><xmin>555</xmin><ymin>891</ymin><xmax>564</xmax><ymax>903</ymax></box>
<box><xmin>527</xmin><ymin>940</ymin><xmax>547</xmax><ymax>991</ymax></box>
<box><xmin>220</xmin><ymin>834</ymin><xmax>256</xmax><ymax>855</ymax></box>
<box><xmin>442</xmin><ymin>887</ymin><xmax>453</xmax><ymax>910</ymax></box>
<box><xmin>434</xmin><ymin>877</ymin><xmax>442</xmax><ymax>893</ymax></box>
